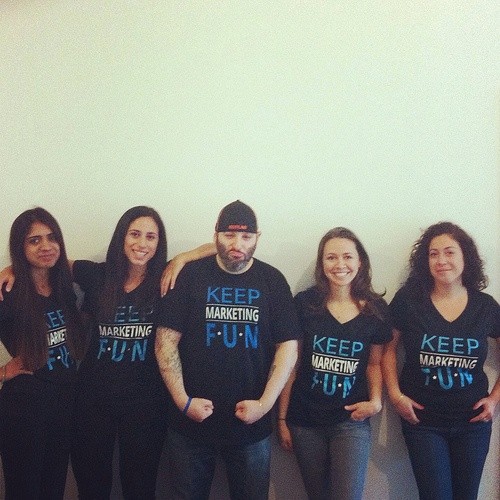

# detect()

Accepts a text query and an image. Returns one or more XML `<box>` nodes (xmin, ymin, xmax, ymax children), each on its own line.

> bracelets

<box><xmin>392</xmin><ymin>394</ymin><xmax>404</xmax><ymax>408</ymax></box>
<box><xmin>278</xmin><ymin>417</ymin><xmax>287</xmax><ymax>420</ymax></box>
<box><xmin>1</xmin><ymin>365</ymin><xmax>7</xmax><ymax>385</ymax></box>
<box><xmin>183</xmin><ymin>396</ymin><xmax>192</xmax><ymax>415</ymax></box>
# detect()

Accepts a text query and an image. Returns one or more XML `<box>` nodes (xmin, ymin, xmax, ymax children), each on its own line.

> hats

<box><xmin>215</xmin><ymin>200</ymin><xmax>257</xmax><ymax>233</ymax></box>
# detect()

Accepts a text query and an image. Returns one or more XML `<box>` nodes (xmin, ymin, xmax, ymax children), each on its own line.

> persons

<box><xmin>0</xmin><ymin>207</ymin><xmax>90</xmax><ymax>500</ymax></box>
<box><xmin>278</xmin><ymin>227</ymin><xmax>394</xmax><ymax>500</ymax></box>
<box><xmin>152</xmin><ymin>200</ymin><xmax>303</xmax><ymax>500</ymax></box>
<box><xmin>0</xmin><ymin>206</ymin><xmax>218</xmax><ymax>500</ymax></box>
<box><xmin>381</xmin><ymin>221</ymin><xmax>500</xmax><ymax>500</ymax></box>
